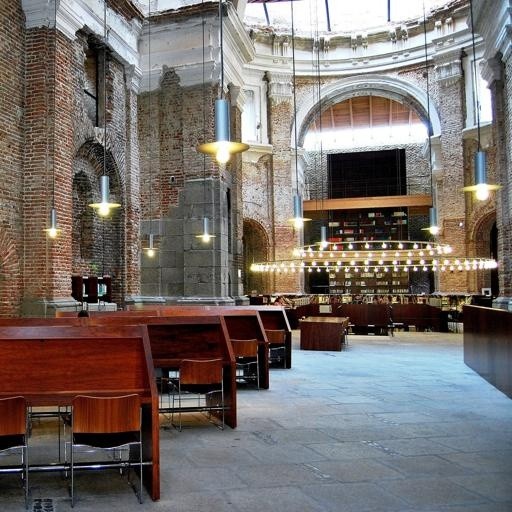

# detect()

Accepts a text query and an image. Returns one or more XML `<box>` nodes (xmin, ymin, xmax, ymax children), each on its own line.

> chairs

<box><xmin>298</xmin><ymin>316</ymin><xmax>349</xmax><ymax>349</ymax></box>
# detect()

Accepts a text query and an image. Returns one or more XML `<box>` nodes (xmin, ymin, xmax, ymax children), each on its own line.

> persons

<box><xmin>273</xmin><ymin>296</ymin><xmax>292</xmax><ymax>307</ymax></box>
